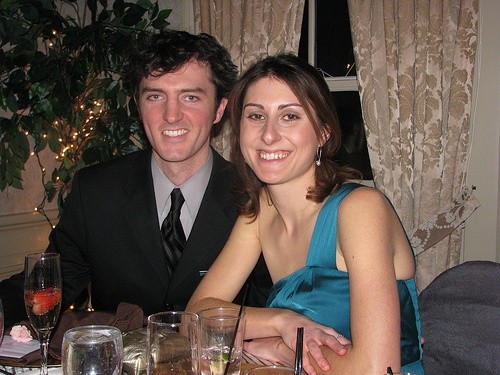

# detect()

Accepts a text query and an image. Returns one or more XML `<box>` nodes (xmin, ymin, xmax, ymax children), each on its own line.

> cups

<box><xmin>196</xmin><ymin>307</ymin><xmax>245</xmax><ymax>375</ymax></box>
<box><xmin>244</xmin><ymin>365</ymin><xmax>305</xmax><ymax>375</ymax></box>
<box><xmin>61</xmin><ymin>325</ymin><xmax>123</xmax><ymax>375</ymax></box>
<box><xmin>146</xmin><ymin>311</ymin><xmax>198</xmax><ymax>375</ymax></box>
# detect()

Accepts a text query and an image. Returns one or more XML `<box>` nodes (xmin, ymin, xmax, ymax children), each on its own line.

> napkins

<box><xmin>0</xmin><ymin>302</ymin><xmax>143</xmax><ymax>366</ymax></box>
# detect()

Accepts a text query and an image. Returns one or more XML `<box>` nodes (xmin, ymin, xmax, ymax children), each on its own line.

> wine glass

<box><xmin>0</xmin><ymin>298</ymin><xmax>4</xmax><ymax>347</ymax></box>
<box><xmin>24</xmin><ymin>252</ymin><xmax>62</xmax><ymax>375</ymax></box>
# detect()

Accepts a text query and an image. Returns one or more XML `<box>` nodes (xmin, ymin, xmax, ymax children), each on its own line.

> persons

<box><xmin>0</xmin><ymin>29</ymin><xmax>275</xmax><ymax>342</ymax></box>
<box><xmin>179</xmin><ymin>53</ymin><xmax>424</xmax><ymax>375</ymax></box>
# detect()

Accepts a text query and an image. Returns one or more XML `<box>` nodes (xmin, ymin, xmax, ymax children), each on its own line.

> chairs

<box><xmin>418</xmin><ymin>261</ymin><xmax>500</xmax><ymax>375</ymax></box>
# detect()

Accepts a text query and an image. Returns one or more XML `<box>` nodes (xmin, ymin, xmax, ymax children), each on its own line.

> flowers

<box><xmin>9</xmin><ymin>325</ymin><xmax>33</xmax><ymax>344</ymax></box>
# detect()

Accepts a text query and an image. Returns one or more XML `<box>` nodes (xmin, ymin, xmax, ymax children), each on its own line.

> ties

<box><xmin>160</xmin><ymin>188</ymin><xmax>187</xmax><ymax>265</ymax></box>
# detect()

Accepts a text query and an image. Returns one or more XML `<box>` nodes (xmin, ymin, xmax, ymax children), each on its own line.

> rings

<box><xmin>336</xmin><ymin>334</ymin><xmax>343</xmax><ymax>340</ymax></box>
